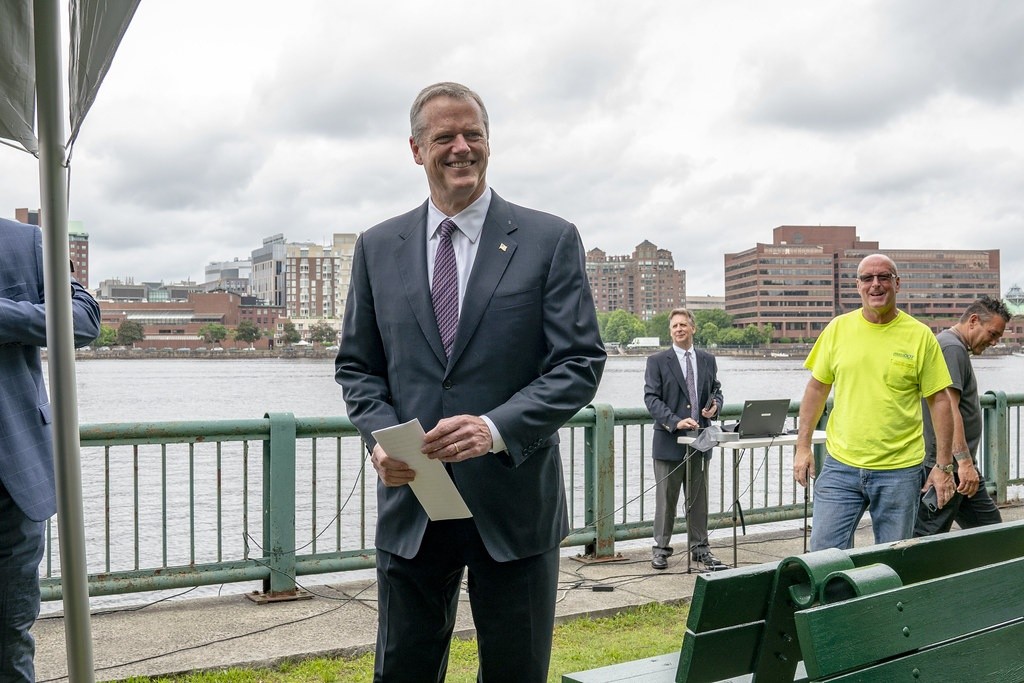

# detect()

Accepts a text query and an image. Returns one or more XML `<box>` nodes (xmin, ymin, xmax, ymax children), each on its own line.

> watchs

<box><xmin>935</xmin><ymin>464</ymin><xmax>953</xmax><ymax>473</ymax></box>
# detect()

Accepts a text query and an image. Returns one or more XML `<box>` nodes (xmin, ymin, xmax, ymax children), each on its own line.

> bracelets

<box><xmin>954</xmin><ymin>451</ymin><xmax>971</xmax><ymax>461</ymax></box>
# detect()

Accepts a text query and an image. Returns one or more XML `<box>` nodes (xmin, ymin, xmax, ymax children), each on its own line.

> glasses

<box><xmin>857</xmin><ymin>272</ymin><xmax>897</xmax><ymax>282</ymax></box>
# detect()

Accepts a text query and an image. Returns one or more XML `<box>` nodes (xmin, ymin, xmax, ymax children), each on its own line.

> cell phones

<box><xmin>922</xmin><ymin>485</ymin><xmax>938</xmax><ymax>511</ymax></box>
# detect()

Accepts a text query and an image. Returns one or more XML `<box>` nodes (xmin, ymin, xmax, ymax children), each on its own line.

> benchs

<box><xmin>561</xmin><ymin>520</ymin><xmax>1024</xmax><ymax>683</ymax></box>
<box><xmin>794</xmin><ymin>553</ymin><xmax>1024</xmax><ymax>683</ymax></box>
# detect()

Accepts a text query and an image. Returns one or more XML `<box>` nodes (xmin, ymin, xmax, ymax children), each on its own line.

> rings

<box><xmin>952</xmin><ymin>490</ymin><xmax>955</xmax><ymax>493</ymax></box>
<box><xmin>454</xmin><ymin>443</ymin><xmax>459</xmax><ymax>453</ymax></box>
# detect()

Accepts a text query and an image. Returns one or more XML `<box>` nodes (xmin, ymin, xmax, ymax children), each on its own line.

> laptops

<box><xmin>737</xmin><ymin>399</ymin><xmax>790</xmax><ymax>439</ymax></box>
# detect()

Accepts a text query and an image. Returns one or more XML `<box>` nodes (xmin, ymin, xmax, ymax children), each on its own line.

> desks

<box><xmin>678</xmin><ymin>429</ymin><xmax>827</xmax><ymax>574</ymax></box>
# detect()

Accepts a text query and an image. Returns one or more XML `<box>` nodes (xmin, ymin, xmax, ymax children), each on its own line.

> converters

<box><xmin>593</xmin><ymin>585</ymin><xmax>614</xmax><ymax>592</ymax></box>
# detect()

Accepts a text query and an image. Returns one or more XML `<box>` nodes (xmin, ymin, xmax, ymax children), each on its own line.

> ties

<box><xmin>431</xmin><ymin>218</ymin><xmax>459</xmax><ymax>362</ymax></box>
<box><xmin>684</xmin><ymin>351</ymin><xmax>700</xmax><ymax>424</ymax></box>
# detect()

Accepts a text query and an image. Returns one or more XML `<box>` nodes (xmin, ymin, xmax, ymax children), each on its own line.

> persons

<box><xmin>0</xmin><ymin>217</ymin><xmax>101</xmax><ymax>683</ymax></box>
<box><xmin>335</xmin><ymin>82</ymin><xmax>608</xmax><ymax>683</ymax></box>
<box><xmin>912</xmin><ymin>296</ymin><xmax>1012</xmax><ymax>539</ymax></box>
<box><xmin>794</xmin><ymin>253</ymin><xmax>955</xmax><ymax>553</ymax></box>
<box><xmin>644</xmin><ymin>308</ymin><xmax>724</xmax><ymax>569</ymax></box>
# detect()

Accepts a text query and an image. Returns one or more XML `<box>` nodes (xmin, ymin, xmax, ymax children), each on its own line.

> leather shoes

<box><xmin>651</xmin><ymin>553</ymin><xmax>668</xmax><ymax>569</ymax></box>
<box><xmin>692</xmin><ymin>551</ymin><xmax>723</xmax><ymax>565</ymax></box>
